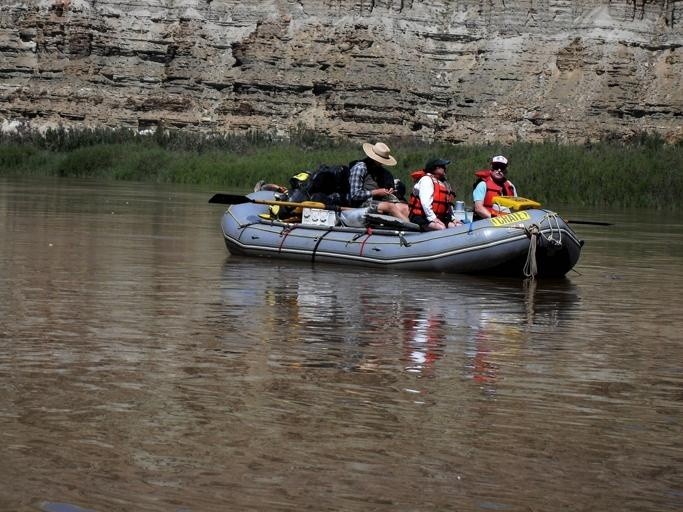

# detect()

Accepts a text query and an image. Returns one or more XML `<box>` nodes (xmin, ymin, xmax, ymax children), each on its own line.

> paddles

<box><xmin>208</xmin><ymin>194</ymin><xmax>355</xmax><ymax>212</ymax></box>
<box><xmin>565</xmin><ymin>219</ymin><xmax>615</xmax><ymax>225</ymax></box>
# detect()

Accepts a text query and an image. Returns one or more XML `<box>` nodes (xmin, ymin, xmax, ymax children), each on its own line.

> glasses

<box><xmin>491</xmin><ymin>164</ymin><xmax>506</xmax><ymax>171</ymax></box>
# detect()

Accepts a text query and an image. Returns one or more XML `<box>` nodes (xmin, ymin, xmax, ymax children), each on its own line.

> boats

<box><xmin>219</xmin><ymin>183</ymin><xmax>586</xmax><ymax>287</ymax></box>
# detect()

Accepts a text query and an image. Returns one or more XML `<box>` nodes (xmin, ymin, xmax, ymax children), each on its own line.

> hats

<box><xmin>425</xmin><ymin>158</ymin><xmax>451</xmax><ymax>173</ymax></box>
<box><xmin>490</xmin><ymin>156</ymin><xmax>509</xmax><ymax>165</ymax></box>
<box><xmin>363</xmin><ymin>142</ymin><xmax>398</xmax><ymax>167</ymax></box>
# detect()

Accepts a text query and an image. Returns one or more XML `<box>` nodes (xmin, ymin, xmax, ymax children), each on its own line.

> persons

<box><xmin>407</xmin><ymin>156</ymin><xmax>463</xmax><ymax>232</ymax></box>
<box><xmin>470</xmin><ymin>154</ymin><xmax>521</xmax><ymax>219</ymax></box>
<box><xmin>340</xmin><ymin>141</ymin><xmax>412</xmax><ymax>224</ymax></box>
